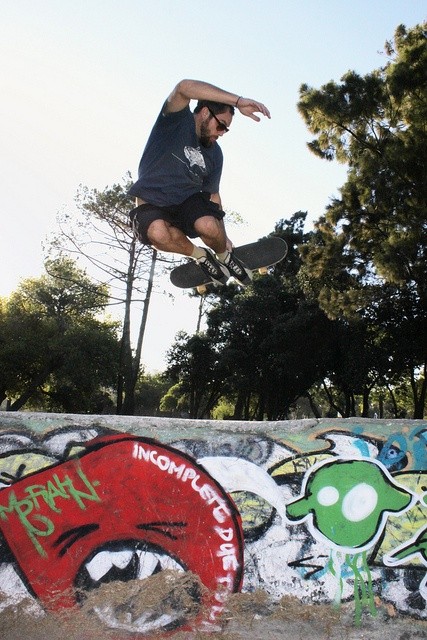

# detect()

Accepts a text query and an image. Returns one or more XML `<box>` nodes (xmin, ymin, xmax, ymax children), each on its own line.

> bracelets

<box><xmin>235</xmin><ymin>96</ymin><xmax>243</xmax><ymax>107</ymax></box>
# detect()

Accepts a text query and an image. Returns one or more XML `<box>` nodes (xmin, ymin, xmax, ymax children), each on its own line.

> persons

<box><xmin>128</xmin><ymin>79</ymin><xmax>271</xmax><ymax>287</ymax></box>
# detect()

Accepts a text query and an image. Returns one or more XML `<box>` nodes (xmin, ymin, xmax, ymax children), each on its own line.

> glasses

<box><xmin>208</xmin><ymin>106</ymin><xmax>231</xmax><ymax>133</ymax></box>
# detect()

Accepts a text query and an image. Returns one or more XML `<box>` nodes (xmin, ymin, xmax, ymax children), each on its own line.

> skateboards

<box><xmin>170</xmin><ymin>237</ymin><xmax>289</xmax><ymax>294</ymax></box>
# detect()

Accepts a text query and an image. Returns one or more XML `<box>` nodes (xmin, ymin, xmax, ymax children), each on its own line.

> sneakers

<box><xmin>212</xmin><ymin>252</ymin><xmax>254</xmax><ymax>288</ymax></box>
<box><xmin>189</xmin><ymin>247</ymin><xmax>232</xmax><ymax>285</ymax></box>
